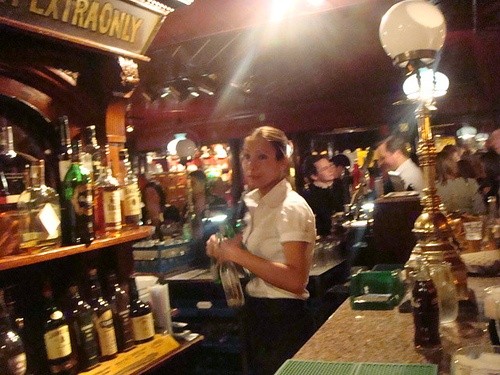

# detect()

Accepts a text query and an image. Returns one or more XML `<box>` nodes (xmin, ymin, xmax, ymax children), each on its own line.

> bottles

<box><xmin>0</xmin><ymin>115</ymin><xmax>145</xmax><ymax>257</ymax></box>
<box><xmin>481</xmin><ymin>196</ymin><xmax>500</xmax><ymax>252</ymax></box>
<box><xmin>411</xmin><ymin>256</ymin><xmax>443</xmax><ymax>353</ymax></box>
<box><xmin>0</xmin><ymin>266</ymin><xmax>155</xmax><ymax>375</ymax></box>
<box><xmin>434</xmin><ymin>263</ymin><xmax>458</xmax><ymax>323</ymax></box>
<box><xmin>207</xmin><ymin>220</ymin><xmax>254</xmax><ymax>307</ymax></box>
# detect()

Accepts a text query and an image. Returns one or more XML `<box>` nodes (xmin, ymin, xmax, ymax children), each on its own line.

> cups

<box><xmin>484</xmin><ymin>286</ymin><xmax>500</xmax><ymax>346</ymax></box>
<box><xmin>450</xmin><ymin>347</ymin><xmax>500</xmax><ymax>375</ymax></box>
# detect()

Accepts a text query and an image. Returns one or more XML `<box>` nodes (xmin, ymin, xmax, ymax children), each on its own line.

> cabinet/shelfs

<box><xmin>0</xmin><ymin>222</ymin><xmax>208</xmax><ymax>375</ymax></box>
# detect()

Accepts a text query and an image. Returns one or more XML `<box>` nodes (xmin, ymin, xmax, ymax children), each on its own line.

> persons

<box><xmin>303</xmin><ymin>155</ymin><xmax>347</xmax><ymax>239</ymax></box>
<box><xmin>204</xmin><ymin>126</ymin><xmax>316</xmax><ymax>375</ymax></box>
<box><xmin>433</xmin><ymin>124</ymin><xmax>499</xmax><ymax>219</ymax></box>
<box><xmin>141</xmin><ymin>184</ymin><xmax>179</xmax><ymax>223</ymax></box>
<box><xmin>377</xmin><ymin>137</ymin><xmax>426</xmax><ymax>193</ymax></box>
<box><xmin>187</xmin><ymin>169</ymin><xmax>218</xmax><ymax>215</ymax></box>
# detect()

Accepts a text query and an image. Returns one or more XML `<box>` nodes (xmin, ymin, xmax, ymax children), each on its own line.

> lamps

<box><xmin>177</xmin><ymin>140</ymin><xmax>198</xmax><ymax>220</ymax></box>
<box><xmin>379</xmin><ymin>0</ymin><xmax>468</xmax><ymax>274</ymax></box>
